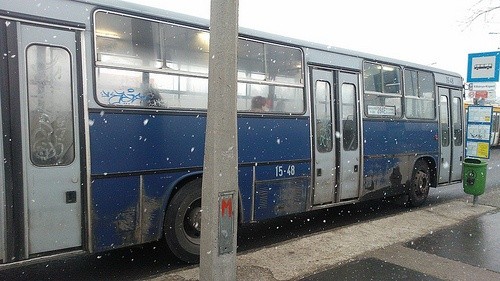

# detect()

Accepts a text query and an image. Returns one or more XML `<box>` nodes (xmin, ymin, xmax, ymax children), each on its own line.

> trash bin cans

<box><xmin>462</xmin><ymin>157</ymin><xmax>488</xmax><ymax>196</ymax></box>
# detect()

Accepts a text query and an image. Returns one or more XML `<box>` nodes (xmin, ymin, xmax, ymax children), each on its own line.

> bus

<box><xmin>0</xmin><ymin>0</ymin><xmax>470</xmax><ymax>275</ymax></box>
